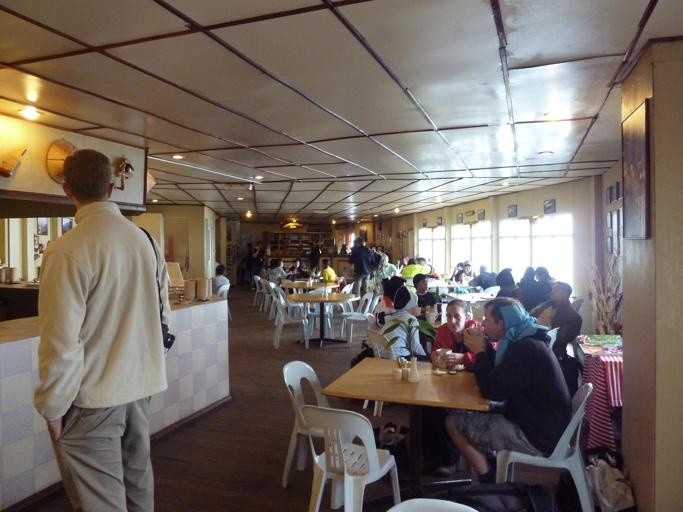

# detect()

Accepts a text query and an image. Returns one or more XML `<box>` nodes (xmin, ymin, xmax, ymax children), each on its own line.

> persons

<box><xmin>211</xmin><ymin>263</ymin><xmax>229</xmax><ymax>294</ymax></box>
<box><xmin>31</xmin><ymin>149</ymin><xmax>177</xmax><ymax>511</ymax></box>
<box><xmin>450</xmin><ymin>260</ymin><xmax>582</xmax><ymax>356</ymax></box>
<box><xmin>268</xmin><ymin>238</ymin><xmax>389</xmax><ymax>307</ymax></box>
<box><xmin>410</xmin><ymin>297</ymin><xmax>572</xmax><ymax>485</ymax></box>
<box><xmin>249</xmin><ymin>241</ymin><xmax>265</xmax><ymax>292</ymax></box>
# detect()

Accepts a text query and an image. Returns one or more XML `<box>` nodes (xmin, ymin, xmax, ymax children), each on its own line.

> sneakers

<box><xmin>436</xmin><ymin>464</ymin><xmax>457</xmax><ymax>476</ymax></box>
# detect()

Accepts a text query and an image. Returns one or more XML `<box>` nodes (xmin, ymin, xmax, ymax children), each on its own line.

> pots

<box><xmin>0</xmin><ymin>267</ymin><xmax>20</xmax><ymax>283</ymax></box>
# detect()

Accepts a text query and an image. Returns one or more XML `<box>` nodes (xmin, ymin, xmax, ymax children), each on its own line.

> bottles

<box><xmin>392</xmin><ymin>357</ymin><xmax>410</xmax><ymax>380</ymax></box>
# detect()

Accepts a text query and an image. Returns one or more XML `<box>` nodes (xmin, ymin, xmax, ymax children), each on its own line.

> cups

<box><xmin>468</xmin><ymin>322</ymin><xmax>482</xmax><ymax>334</ymax></box>
<box><xmin>410</xmin><ymin>304</ymin><xmax>438</xmax><ymax>325</ymax></box>
<box><xmin>183</xmin><ymin>276</ymin><xmax>212</xmax><ymax>302</ymax></box>
<box><xmin>436</xmin><ymin>348</ymin><xmax>452</xmax><ymax>369</ymax></box>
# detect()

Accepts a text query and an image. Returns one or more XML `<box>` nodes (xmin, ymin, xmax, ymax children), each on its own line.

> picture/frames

<box><xmin>616</xmin><ymin>96</ymin><xmax>653</xmax><ymax>242</ymax></box>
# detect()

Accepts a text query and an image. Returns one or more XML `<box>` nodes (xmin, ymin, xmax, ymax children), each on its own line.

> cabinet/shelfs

<box><xmin>264</xmin><ymin>230</ymin><xmax>336</xmax><ymax>259</ymax></box>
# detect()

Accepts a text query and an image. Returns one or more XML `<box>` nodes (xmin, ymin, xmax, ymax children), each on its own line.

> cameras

<box><xmin>162</xmin><ymin>323</ymin><xmax>176</xmax><ymax>348</ymax></box>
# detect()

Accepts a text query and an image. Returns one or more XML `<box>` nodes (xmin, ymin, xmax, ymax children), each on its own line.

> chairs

<box><xmin>216</xmin><ymin>283</ymin><xmax>234</xmax><ymax>324</ymax></box>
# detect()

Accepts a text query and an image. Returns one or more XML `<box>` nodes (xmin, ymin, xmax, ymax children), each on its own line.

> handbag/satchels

<box><xmin>373</xmin><ymin>421</ymin><xmax>414</xmax><ymax>474</ymax></box>
